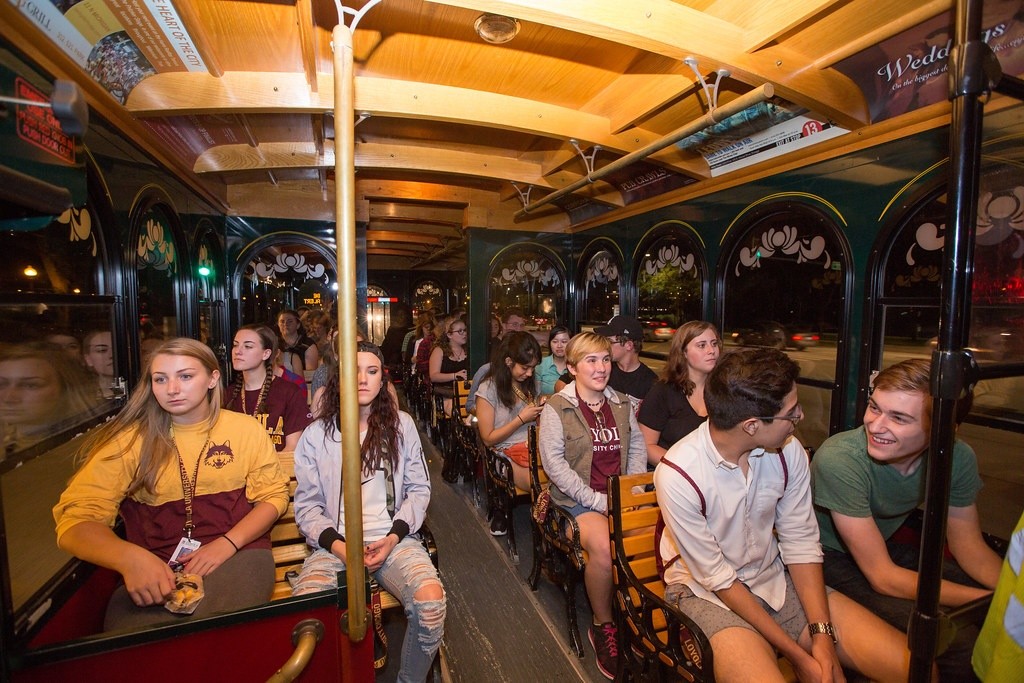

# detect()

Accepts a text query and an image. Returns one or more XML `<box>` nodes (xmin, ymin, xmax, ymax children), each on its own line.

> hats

<box><xmin>593</xmin><ymin>314</ymin><xmax>644</xmax><ymax>340</ymax></box>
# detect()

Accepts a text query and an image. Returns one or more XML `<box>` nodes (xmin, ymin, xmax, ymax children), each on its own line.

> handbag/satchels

<box><xmin>442</xmin><ymin>449</ymin><xmax>471</xmax><ymax>482</ymax></box>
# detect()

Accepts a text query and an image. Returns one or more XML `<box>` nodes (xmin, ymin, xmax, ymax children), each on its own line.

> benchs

<box><xmin>483</xmin><ymin>444</ymin><xmax>526</xmax><ymax>565</ymax></box>
<box><xmin>606</xmin><ymin>472</ymin><xmax>713</xmax><ymax>683</ymax></box>
<box><xmin>527</xmin><ymin>424</ymin><xmax>589</xmax><ymax>658</ymax></box>
<box><xmin>269</xmin><ymin>452</ymin><xmax>442</xmax><ymax>682</ymax></box>
<box><xmin>452</xmin><ymin>379</ymin><xmax>479</xmax><ymax>509</ymax></box>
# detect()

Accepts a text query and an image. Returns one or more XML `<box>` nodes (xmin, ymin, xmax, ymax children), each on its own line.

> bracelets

<box><xmin>454</xmin><ymin>373</ymin><xmax>455</xmax><ymax>380</ymax></box>
<box><xmin>223</xmin><ymin>535</ymin><xmax>239</xmax><ymax>551</ymax></box>
<box><xmin>518</xmin><ymin>415</ymin><xmax>525</xmax><ymax>425</ymax></box>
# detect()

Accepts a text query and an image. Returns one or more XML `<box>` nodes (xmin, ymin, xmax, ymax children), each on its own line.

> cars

<box><xmin>921</xmin><ymin>320</ymin><xmax>1024</xmax><ymax>365</ymax></box>
<box><xmin>638</xmin><ymin>316</ymin><xmax>675</xmax><ymax>343</ymax></box>
<box><xmin>730</xmin><ymin>319</ymin><xmax>821</xmax><ymax>351</ymax></box>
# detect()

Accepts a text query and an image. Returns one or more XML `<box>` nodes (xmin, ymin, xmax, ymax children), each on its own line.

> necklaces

<box><xmin>585</xmin><ymin>399</ymin><xmax>604</xmax><ymax>407</ymax></box>
<box><xmin>454</xmin><ymin>348</ymin><xmax>462</xmax><ymax>359</ymax></box>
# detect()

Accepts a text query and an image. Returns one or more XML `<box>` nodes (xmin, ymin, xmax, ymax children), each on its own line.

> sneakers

<box><xmin>490</xmin><ymin>511</ymin><xmax>507</xmax><ymax>535</ymax></box>
<box><xmin>588</xmin><ymin>617</ymin><xmax>633</xmax><ymax>680</ymax></box>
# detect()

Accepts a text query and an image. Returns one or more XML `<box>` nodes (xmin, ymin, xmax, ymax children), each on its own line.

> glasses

<box><xmin>506</xmin><ymin>322</ymin><xmax>525</xmax><ymax>328</ymax></box>
<box><xmin>450</xmin><ymin>328</ymin><xmax>468</xmax><ymax>335</ymax></box>
<box><xmin>753</xmin><ymin>404</ymin><xmax>802</xmax><ymax>426</ymax></box>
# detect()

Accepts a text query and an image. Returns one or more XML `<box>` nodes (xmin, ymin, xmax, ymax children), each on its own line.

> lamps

<box><xmin>474</xmin><ymin>12</ymin><xmax>522</xmax><ymax>44</ymax></box>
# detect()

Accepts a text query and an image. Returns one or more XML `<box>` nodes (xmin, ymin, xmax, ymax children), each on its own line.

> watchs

<box><xmin>808</xmin><ymin>623</ymin><xmax>840</xmax><ymax>644</ymax></box>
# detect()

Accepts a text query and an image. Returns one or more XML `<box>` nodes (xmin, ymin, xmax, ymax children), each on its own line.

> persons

<box><xmin>970</xmin><ymin>510</ymin><xmax>1024</xmax><ymax>683</ymax></box>
<box><xmin>402</xmin><ymin>310</ymin><xmax>468</xmax><ymax>439</ymax></box>
<box><xmin>636</xmin><ymin>320</ymin><xmax>723</xmax><ymax>507</ymax></box>
<box><xmin>265</xmin><ymin>306</ymin><xmax>399</xmax><ymax>418</ymax></box>
<box><xmin>652</xmin><ymin>350</ymin><xmax>939</xmax><ymax>683</ymax></box>
<box><xmin>0</xmin><ymin>329</ymin><xmax>114</xmax><ymax>452</ymax></box>
<box><xmin>538</xmin><ymin>334</ymin><xmax>659</xmax><ymax>681</ymax></box>
<box><xmin>465</xmin><ymin>308</ymin><xmax>571</xmax><ymax>535</ymax></box>
<box><xmin>293</xmin><ymin>340</ymin><xmax>447</xmax><ymax>683</ymax></box>
<box><xmin>52</xmin><ymin>337</ymin><xmax>291</xmax><ymax>633</ymax></box>
<box><xmin>142</xmin><ymin>339</ymin><xmax>162</xmax><ymax>357</ymax></box>
<box><xmin>181</xmin><ymin>551</ymin><xmax>189</xmax><ymax>558</ymax></box>
<box><xmin>809</xmin><ymin>359</ymin><xmax>1005</xmax><ymax>683</ymax></box>
<box><xmin>220</xmin><ymin>324</ymin><xmax>313</xmax><ymax>452</ymax></box>
<box><xmin>554</xmin><ymin>314</ymin><xmax>661</xmax><ymax>420</ymax></box>
